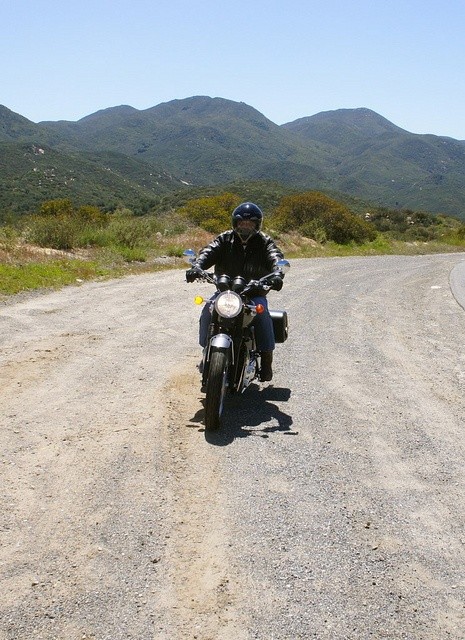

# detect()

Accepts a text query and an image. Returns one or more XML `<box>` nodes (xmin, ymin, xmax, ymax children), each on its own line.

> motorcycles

<box><xmin>182</xmin><ymin>249</ymin><xmax>288</xmax><ymax>432</ymax></box>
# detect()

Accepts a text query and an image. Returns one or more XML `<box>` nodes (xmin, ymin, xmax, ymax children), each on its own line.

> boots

<box><xmin>260</xmin><ymin>352</ymin><xmax>273</xmax><ymax>381</ymax></box>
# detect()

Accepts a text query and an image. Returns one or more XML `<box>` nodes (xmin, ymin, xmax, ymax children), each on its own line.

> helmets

<box><xmin>231</xmin><ymin>202</ymin><xmax>262</xmax><ymax>235</ymax></box>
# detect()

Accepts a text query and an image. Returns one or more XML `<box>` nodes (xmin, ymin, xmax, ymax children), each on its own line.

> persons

<box><xmin>186</xmin><ymin>202</ymin><xmax>284</xmax><ymax>381</ymax></box>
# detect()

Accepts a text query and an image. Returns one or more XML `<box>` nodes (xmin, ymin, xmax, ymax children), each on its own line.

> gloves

<box><xmin>186</xmin><ymin>267</ymin><xmax>201</xmax><ymax>283</ymax></box>
<box><xmin>270</xmin><ymin>276</ymin><xmax>283</xmax><ymax>291</ymax></box>
